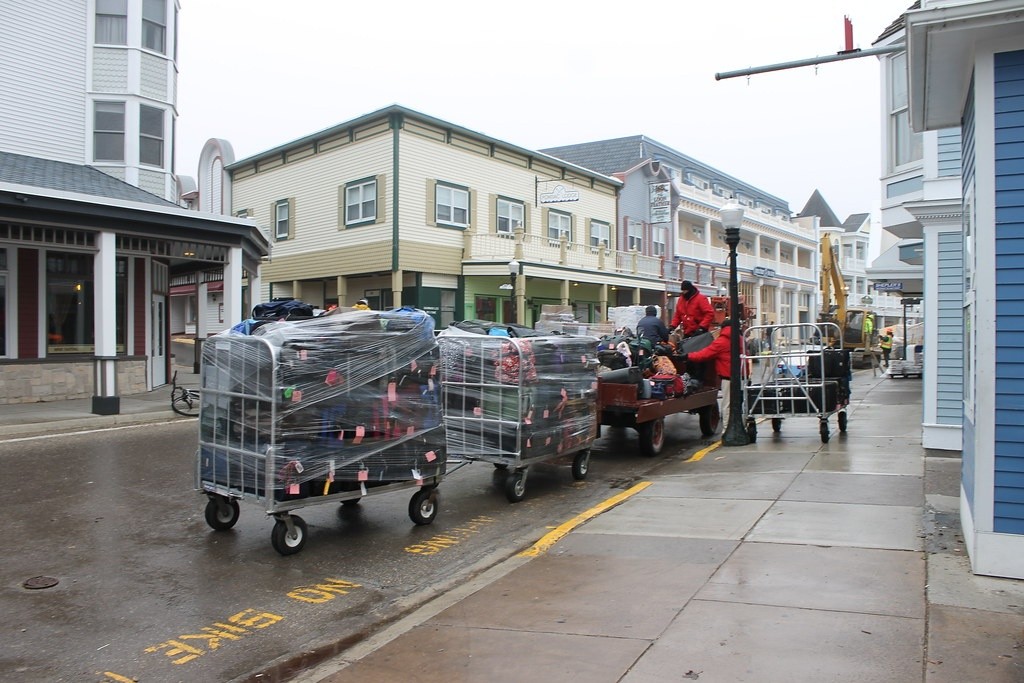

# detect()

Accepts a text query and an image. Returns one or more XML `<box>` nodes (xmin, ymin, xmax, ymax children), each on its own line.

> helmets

<box><xmin>886</xmin><ymin>329</ymin><xmax>893</xmax><ymax>332</ymax></box>
<box><xmin>868</xmin><ymin>315</ymin><xmax>874</xmax><ymax>319</ymax></box>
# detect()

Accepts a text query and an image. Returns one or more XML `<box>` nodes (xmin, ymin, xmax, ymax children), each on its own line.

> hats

<box><xmin>360</xmin><ymin>298</ymin><xmax>368</xmax><ymax>305</ymax></box>
<box><xmin>722</xmin><ymin>320</ymin><xmax>731</xmax><ymax>326</ymax></box>
<box><xmin>681</xmin><ymin>281</ymin><xmax>696</xmax><ymax>300</ymax></box>
<box><xmin>646</xmin><ymin>306</ymin><xmax>657</xmax><ymax>314</ymax></box>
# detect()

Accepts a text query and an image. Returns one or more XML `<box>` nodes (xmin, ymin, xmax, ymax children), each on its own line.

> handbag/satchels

<box><xmin>252</xmin><ymin>300</ymin><xmax>314</xmax><ymax>318</ymax></box>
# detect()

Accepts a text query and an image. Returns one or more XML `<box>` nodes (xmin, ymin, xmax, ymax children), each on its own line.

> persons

<box><xmin>877</xmin><ymin>327</ymin><xmax>894</xmax><ymax>367</ymax></box>
<box><xmin>766</xmin><ymin>321</ymin><xmax>774</xmax><ymax>350</ymax></box>
<box><xmin>679</xmin><ymin>319</ymin><xmax>750</xmax><ymax>434</ymax></box>
<box><xmin>668</xmin><ymin>280</ymin><xmax>715</xmax><ymax>389</ymax></box>
<box><xmin>636</xmin><ymin>306</ymin><xmax>670</xmax><ymax>346</ymax></box>
<box><xmin>352</xmin><ymin>298</ymin><xmax>371</xmax><ymax>310</ymax></box>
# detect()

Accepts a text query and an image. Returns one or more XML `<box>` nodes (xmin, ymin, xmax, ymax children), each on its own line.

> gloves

<box><xmin>695</xmin><ymin>328</ymin><xmax>705</xmax><ymax>335</ymax></box>
<box><xmin>667</xmin><ymin>327</ymin><xmax>674</xmax><ymax>332</ymax></box>
<box><xmin>679</xmin><ymin>354</ymin><xmax>687</xmax><ymax>360</ymax></box>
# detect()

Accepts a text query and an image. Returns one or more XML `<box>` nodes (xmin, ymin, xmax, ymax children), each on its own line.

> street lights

<box><xmin>508</xmin><ymin>257</ymin><xmax>520</xmax><ymax>323</ymax></box>
<box><xmin>718</xmin><ymin>197</ymin><xmax>752</xmax><ymax>446</ymax></box>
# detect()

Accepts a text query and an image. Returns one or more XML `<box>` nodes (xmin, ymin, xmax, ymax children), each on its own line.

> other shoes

<box><xmin>883</xmin><ymin>364</ymin><xmax>889</xmax><ymax>367</ymax></box>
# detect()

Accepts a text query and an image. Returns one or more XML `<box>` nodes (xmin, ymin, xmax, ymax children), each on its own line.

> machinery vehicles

<box><xmin>809</xmin><ymin>232</ymin><xmax>881</xmax><ymax>370</ymax></box>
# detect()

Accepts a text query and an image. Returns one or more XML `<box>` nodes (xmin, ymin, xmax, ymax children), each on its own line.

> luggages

<box><xmin>436</xmin><ymin>337</ymin><xmax>598</xmax><ymax>459</ymax></box>
<box><xmin>202</xmin><ymin>308</ymin><xmax>447</xmax><ymax>502</ymax></box>
<box><xmin>598</xmin><ymin>326</ymin><xmax>714</xmax><ymax>400</ymax></box>
